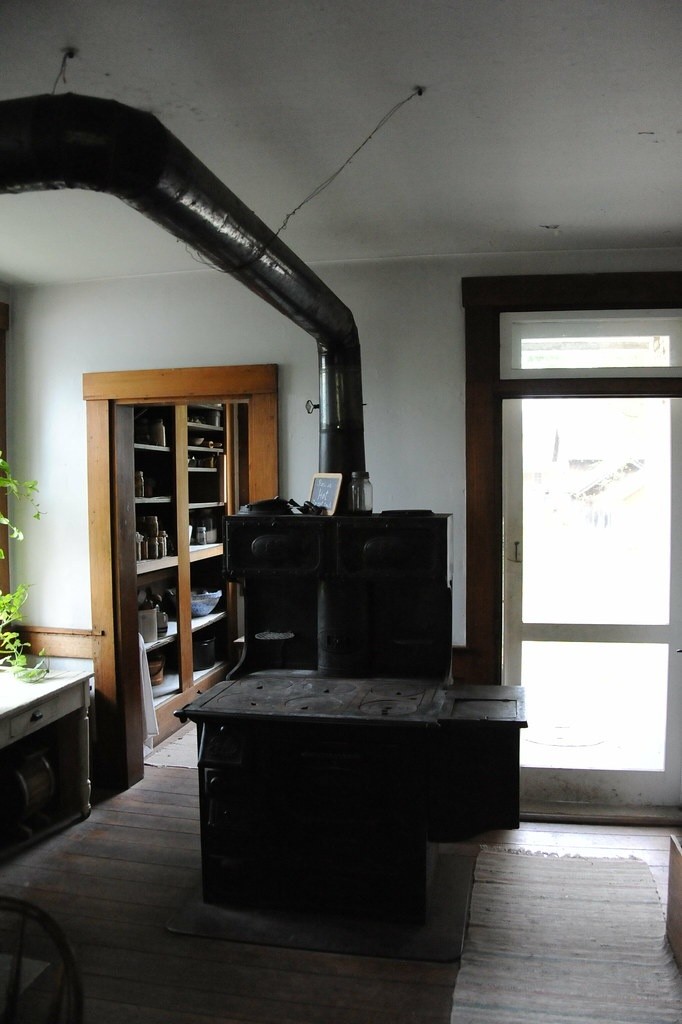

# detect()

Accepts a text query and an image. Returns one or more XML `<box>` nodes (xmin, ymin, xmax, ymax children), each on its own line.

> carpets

<box><xmin>451</xmin><ymin>846</ymin><xmax>682</xmax><ymax>1024</ymax></box>
<box><xmin>165</xmin><ymin>843</ymin><xmax>478</xmax><ymax>962</ymax></box>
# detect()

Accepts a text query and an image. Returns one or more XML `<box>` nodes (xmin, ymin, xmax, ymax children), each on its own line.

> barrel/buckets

<box><xmin>188</xmin><ymin>634</ymin><xmax>216</xmax><ymax>671</ymax></box>
<box><xmin>148</xmin><ymin>656</ymin><xmax>165</xmax><ymax>686</ymax></box>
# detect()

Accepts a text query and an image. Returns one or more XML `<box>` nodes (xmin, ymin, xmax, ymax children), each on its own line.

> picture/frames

<box><xmin>308</xmin><ymin>473</ymin><xmax>343</xmax><ymax>516</ymax></box>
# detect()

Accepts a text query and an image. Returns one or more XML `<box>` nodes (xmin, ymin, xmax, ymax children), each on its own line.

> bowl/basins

<box><xmin>168</xmin><ymin>587</ymin><xmax>223</xmax><ymax>618</ymax></box>
<box><xmin>193</xmin><ymin>438</ymin><xmax>204</xmax><ymax>445</ymax></box>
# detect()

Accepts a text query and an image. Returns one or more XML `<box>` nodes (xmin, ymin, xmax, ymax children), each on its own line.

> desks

<box><xmin>0</xmin><ymin>665</ymin><xmax>94</xmax><ymax>818</ymax></box>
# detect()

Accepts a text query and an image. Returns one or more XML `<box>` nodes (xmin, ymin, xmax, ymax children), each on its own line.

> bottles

<box><xmin>349</xmin><ymin>471</ymin><xmax>374</xmax><ymax>515</ymax></box>
<box><xmin>196</xmin><ymin>521</ymin><xmax>207</xmax><ymax>544</ymax></box>
<box><xmin>153</xmin><ymin>419</ymin><xmax>167</xmax><ymax>447</ymax></box>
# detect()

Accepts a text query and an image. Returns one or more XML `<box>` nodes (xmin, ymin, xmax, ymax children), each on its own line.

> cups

<box><xmin>158</xmin><ymin>612</ymin><xmax>168</xmax><ymax>636</ymax></box>
<box><xmin>206</xmin><ymin>529</ymin><xmax>220</xmax><ymax>543</ymax></box>
<box><xmin>138</xmin><ymin>609</ymin><xmax>158</xmax><ymax>643</ymax></box>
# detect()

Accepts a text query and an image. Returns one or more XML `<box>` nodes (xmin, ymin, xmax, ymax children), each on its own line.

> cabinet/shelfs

<box><xmin>111</xmin><ymin>402</ymin><xmax>240</xmax><ymax>749</ymax></box>
